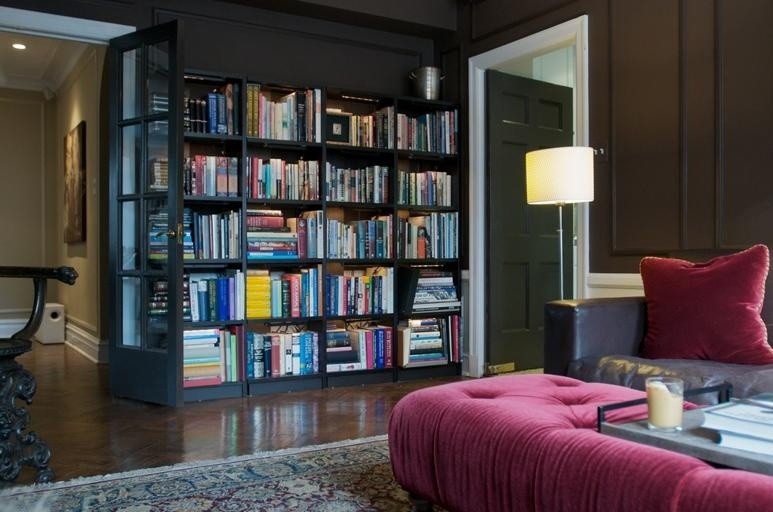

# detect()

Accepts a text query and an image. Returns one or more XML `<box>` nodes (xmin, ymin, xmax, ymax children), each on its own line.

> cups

<box><xmin>645</xmin><ymin>376</ymin><xmax>684</xmax><ymax>435</ymax></box>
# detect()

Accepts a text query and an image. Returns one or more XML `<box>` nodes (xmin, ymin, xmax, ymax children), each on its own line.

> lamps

<box><xmin>524</xmin><ymin>146</ymin><xmax>595</xmax><ymax>300</ymax></box>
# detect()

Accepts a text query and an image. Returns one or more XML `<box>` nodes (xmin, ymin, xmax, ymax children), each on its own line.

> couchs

<box><xmin>542</xmin><ymin>247</ymin><xmax>773</xmax><ymax>398</ymax></box>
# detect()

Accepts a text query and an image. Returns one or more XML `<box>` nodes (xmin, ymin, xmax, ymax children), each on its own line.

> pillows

<box><xmin>638</xmin><ymin>242</ymin><xmax>773</xmax><ymax>365</ymax></box>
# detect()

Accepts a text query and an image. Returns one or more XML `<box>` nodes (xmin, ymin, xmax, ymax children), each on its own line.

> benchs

<box><xmin>387</xmin><ymin>373</ymin><xmax>773</xmax><ymax>512</ymax></box>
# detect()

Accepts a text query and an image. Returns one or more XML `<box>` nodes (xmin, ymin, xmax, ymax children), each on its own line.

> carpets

<box><xmin>0</xmin><ymin>432</ymin><xmax>453</xmax><ymax>512</ymax></box>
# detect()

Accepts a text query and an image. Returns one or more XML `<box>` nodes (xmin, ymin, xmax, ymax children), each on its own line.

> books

<box><xmin>714</xmin><ymin>431</ymin><xmax>772</xmax><ymax>457</ymax></box>
<box><xmin>698</xmin><ymin>389</ymin><xmax>772</xmax><ymax>442</ymax></box>
<box><xmin>144</xmin><ymin>75</ymin><xmax>467</xmax><ymax>392</ymax></box>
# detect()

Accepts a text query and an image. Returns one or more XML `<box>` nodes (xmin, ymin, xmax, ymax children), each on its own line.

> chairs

<box><xmin>1</xmin><ymin>263</ymin><xmax>79</xmax><ymax>494</ymax></box>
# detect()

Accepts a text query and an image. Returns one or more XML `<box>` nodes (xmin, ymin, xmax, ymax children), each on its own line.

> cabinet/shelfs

<box><xmin>134</xmin><ymin>66</ymin><xmax>469</xmax><ymax>404</ymax></box>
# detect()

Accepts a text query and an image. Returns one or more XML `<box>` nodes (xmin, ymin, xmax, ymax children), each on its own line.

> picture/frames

<box><xmin>61</xmin><ymin>120</ymin><xmax>87</xmax><ymax>244</ymax></box>
